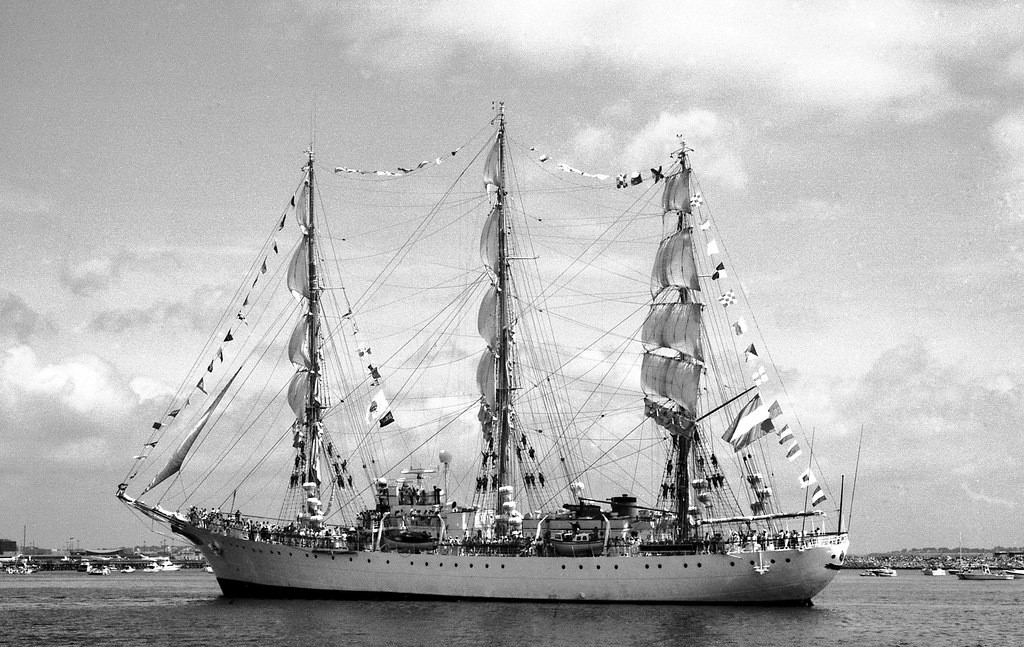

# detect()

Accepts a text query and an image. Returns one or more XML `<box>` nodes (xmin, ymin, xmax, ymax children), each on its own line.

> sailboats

<box><xmin>116</xmin><ymin>99</ymin><xmax>865</xmax><ymax>604</ymax></box>
<box><xmin>5</xmin><ymin>526</ymin><xmax>39</xmax><ymax>576</ymax></box>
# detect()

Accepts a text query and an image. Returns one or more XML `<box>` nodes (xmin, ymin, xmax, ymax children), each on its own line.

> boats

<box><xmin>1006</xmin><ymin>567</ymin><xmax>1024</xmax><ymax>575</ymax></box>
<box><xmin>948</xmin><ymin>569</ymin><xmax>964</xmax><ymax>575</ymax></box>
<box><xmin>956</xmin><ymin>563</ymin><xmax>1015</xmax><ymax>580</ymax></box>
<box><xmin>78</xmin><ymin>558</ymin><xmax>90</xmax><ymax>571</ymax></box>
<box><xmin>161</xmin><ymin>560</ymin><xmax>183</xmax><ymax>572</ymax></box>
<box><xmin>923</xmin><ymin>558</ymin><xmax>946</xmax><ymax>575</ymax></box>
<box><xmin>858</xmin><ymin>561</ymin><xmax>894</xmax><ymax>576</ymax></box>
<box><xmin>88</xmin><ymin>565</ymin><xmax>112</xmax><ymax>576</ymax></box>
<box><xmin>205</xmin><ymin>563</ymin><xmax>214</xmax><ymax>573</ymax></box>
<box><xmin>121</xmin><ymin>564</ymin><xmax>135</xmax><ymax>572</ymax></box>
<box><xmin>142</xmin><ymin>561</ymin><xmax>162</xmax><ymax>572</ymax></box>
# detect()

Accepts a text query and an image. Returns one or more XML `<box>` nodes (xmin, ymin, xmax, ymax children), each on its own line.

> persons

<box><xmin>347</xmin><ymin>482</ymin><xmax>441</xmax><ymax>551</ymax></box>
<box><xmin>661</xmin><ymin>459</ymin><xmax>675</xmax><ymax>500</ymax></box>
<box><xmin>476</xmin><ymin>433</ymin><xmax>544</xmax><ymax>494</ymax></box>
<box><xmin>698</xmin><ymin>454</ymin><xmax>725</xmax><ymax>489</ymax></box>
<box><xmin>746</xmin><ymin>473</ymin><xmax>771</xmax><ymax>515</ymax></box>
<box><xmin>436</xmin><ymin>527</ymin><xmax>821</xmax><ymax>556</ymax></box>
<box><xmin>187</xmin><ymin>504</ymin><xmax>347</xmax><ymax>549</ymax></box>
<box><xmin>289</xmin><ymin>430</ymin><xmax>353</xmax><ymax>489</ymax></box>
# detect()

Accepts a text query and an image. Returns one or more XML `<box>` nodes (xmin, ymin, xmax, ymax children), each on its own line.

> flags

<box><xmin>786</xmin><ymin>441</ymin><xmax>802</xmax><ymax>461</ymax></box>
<box><xmin>775</xmin><ymin>425</ymin><xmax>794</xmax><ymax>445</ymax></box>
<box><xmin>129</xmin><ymin>195</ymin><xmax>295</xmax><ymax>480</ymax></box>
<box><xmin>799</xmin><ymin>467</ymin><xmax>816</xmax><ymax>488</ymax></box>
<box><xmin>341</xmin><ymin>309</ymin><xmax>395</xmax><ymax>428</ymax></box>
<box><xmin>768</xmin><ymin>400</ymin><xmax>782</xmax><ymax>419</ymax></box>
<box><xmin>722</xmin><ymin>394</ymin><xmax>776</xmax><ymax>453</ymax></box>
<box><xmin>812</xmin><ymin>486</ymin><xmax>827</xmax><ymax>507</ymax></box>
<box><xmin>539</xmin><ymin>153</ymin><xmax>664</xmax><ymax>188</ymax></box>
<box><xmin>335</xmin><ymin>147</ymin><xmax>461</xmax><ymax>177</ymax></box>
<box><xmin>690</xmin><ymin>191</ymin><xmax>769</xmax><ymax>385</ymax></box>
